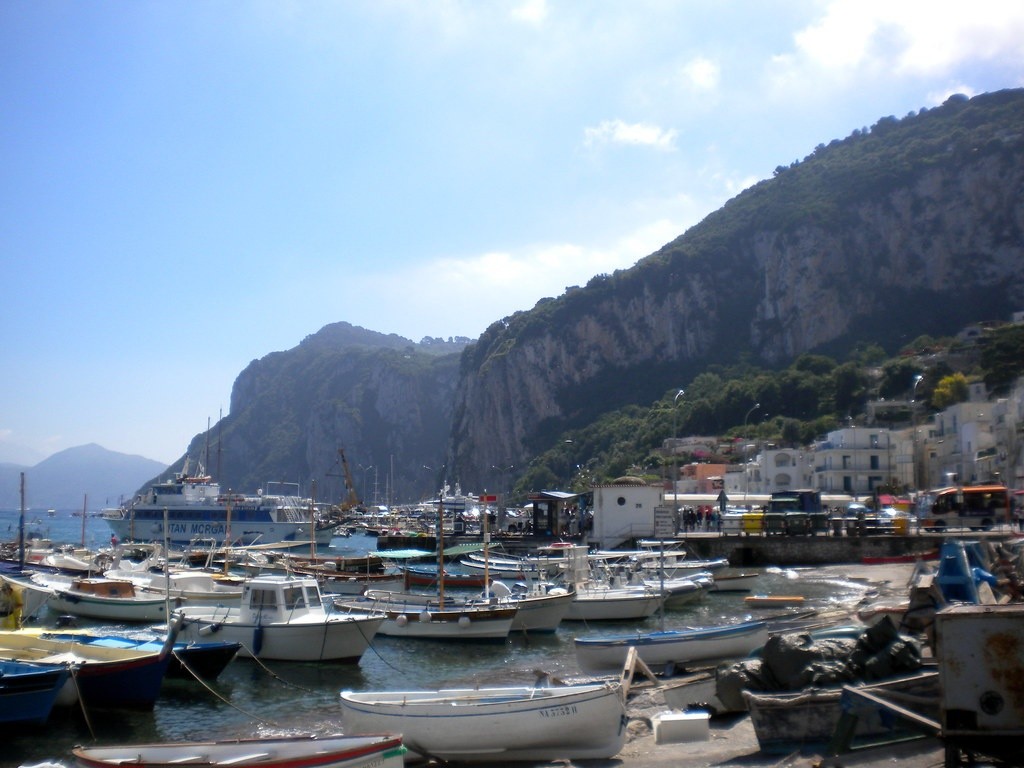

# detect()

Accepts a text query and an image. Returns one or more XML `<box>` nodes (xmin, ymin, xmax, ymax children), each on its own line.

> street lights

<box><xmin>912</xmin><ymin>375</ymin><xmax>923</xmax><ymax>532</ymax></box>
<box><xmin>673</xmin><ymin>389</ymin><xmax>685</xmax><ymax>536</ymax></box>
<box><xmin>744</xmin><ymin>404</ymin><xmax>760</xmax><ymax>509</ymax></box>
<box><xmin>492</xmin><ymin>464</ymin><xmax>513</xmax><ymax>533</ymax></box>
<box><xmin>358</xmin><ymin>464</ymin><xmax>373</xmax><ymax>511</ymax></box>
<box><xmin>422</xmin><ymin>465</ymin><xmax>435</xmax><ymax>509</ymax></box>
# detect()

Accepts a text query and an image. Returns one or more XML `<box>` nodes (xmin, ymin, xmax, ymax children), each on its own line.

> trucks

<box><xmin>766</xmin><ymin>489</ymin><xmax>824</xmax><ymax>536</ymax></box>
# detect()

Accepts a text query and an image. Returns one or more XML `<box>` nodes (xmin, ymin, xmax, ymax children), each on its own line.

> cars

<box><xmin>896</xmin><ymin>511</ymin><xmax>918</xmax><ymax>526</ymax></box>
<box><xmin>847</xmin><ymin>502</ymin><xmax>896</xmax><ymax>534</ymax></box>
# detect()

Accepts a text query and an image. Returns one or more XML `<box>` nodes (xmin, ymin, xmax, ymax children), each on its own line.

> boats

<box><xmin>740</xmin><ymin>672</ymin><xmax>942</xmax><ymax>751</ymax></box>
<box><xmin>72</xmin><ymin>732</ymin><xmax>407</xmax><ymax>768</ymax></box>
<box><xmin>574</xmin><ymin>609</ymin><xmax>818</xmax><ymax>670</ymax></box>
<box><xmin>0</xmin><ymin>516</ymin><xmax>733</xmax><ymax>712</ymax></box>
<box><xmin>707</xmin><ymin>573</ymin><xmax>762</xmax><ymax>591</ymax></box>
<box><xmin>339</xmin><ymin>684</ymin><xmax>625</xmax><ymax>763</ymax></box>
<box><xmin>857</xmin><ymin>598</ymin><xmax>913</xmax><ymax>626</ymax></box>
<box><xmin>745</xmin><ymin>595</ymin><xmax>804</xmax><ymax>607</ymax></box>
<box><xmin>745</xmin><ymin>623</ymin><xmax>923</xmax><ymax>663</ymax></box>
<box><xmin>101</xmin><ymin>406</ymin><xmax>358</xmax><ymax>547</ymax></box>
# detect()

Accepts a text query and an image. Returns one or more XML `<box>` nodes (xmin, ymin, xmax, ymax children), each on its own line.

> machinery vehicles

<box><xmin>334</xmin><ymin>446</ymin><xmax>359</xmax><ymax>510</ymax></box>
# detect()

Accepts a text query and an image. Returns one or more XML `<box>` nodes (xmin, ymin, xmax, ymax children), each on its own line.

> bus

<box><xmin>919</xmin><ymin>486</ymin><xmax>1009</xmax><ymax>531</ymax></box>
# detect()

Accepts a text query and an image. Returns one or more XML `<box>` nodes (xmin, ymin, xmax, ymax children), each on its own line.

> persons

<box><xmin>487</xmin><ymin>505</ymin><xmax>723</xmax><ymax>534</ymax></box>
<box><xmin>111</xmin><ymin>533</ymin><xmax>118</xmax><ymax>550</ymax></box>
<box><xmin>0</xmin><ymin>583</ymin><xmax>24</xmax><ymax>630</ymax></box>
<box><xmin>257</xmin><ymin>487</ymin><xmax>262</xmax><ymax>497</ymax></box>
